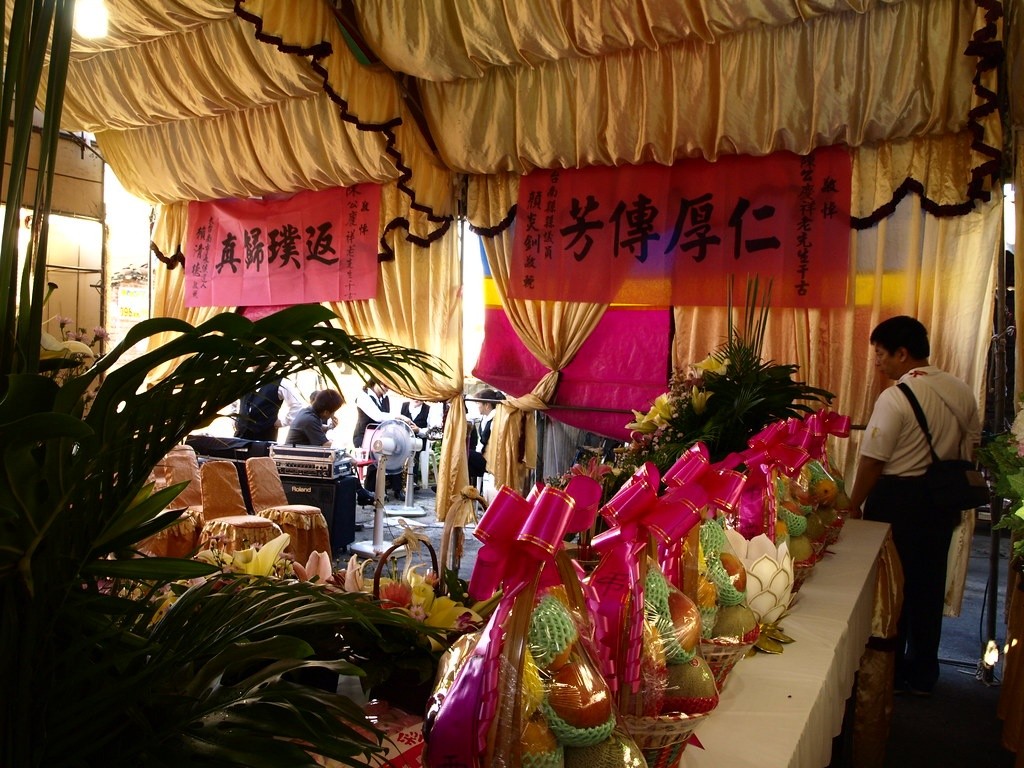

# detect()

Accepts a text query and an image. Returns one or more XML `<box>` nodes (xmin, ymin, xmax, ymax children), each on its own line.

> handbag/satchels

<box><xmin>928</xmin><ymin>460</ymin><xmax>990</xmax><ymax>511</ymax></box>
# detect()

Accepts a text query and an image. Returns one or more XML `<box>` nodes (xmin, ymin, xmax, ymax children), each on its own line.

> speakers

<box><xmin>280</xmin><ymin>475</ymin><xmax>357</xmax><ymax>552</ymax></box>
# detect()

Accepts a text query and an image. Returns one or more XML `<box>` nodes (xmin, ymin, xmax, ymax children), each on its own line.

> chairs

<box><xmin>129</xmin><ymin>443</ymin><xmax>332</xmax><ymax>574</ymax></box>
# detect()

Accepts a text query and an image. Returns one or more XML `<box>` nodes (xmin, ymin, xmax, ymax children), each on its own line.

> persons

<box><xmin>285</xmin><ymin>376</ymin><xmax>506</xmax><ymax>529</ymax></box>
<box><xmin>233</xmin><ymin>354</ymin><xmax>302</xmax><ymax>445</ymax></box>
<box><xmin>846</xmin><ymin>315</ymin><xmax>982</xmax><ymax>696</ymax></box>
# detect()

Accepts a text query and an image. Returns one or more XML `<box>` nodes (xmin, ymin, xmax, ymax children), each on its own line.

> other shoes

<box><xmin>356</xmin><ymin>524</ymin><xmax>364</xmax><ymax>529</ymax></box>
<box><xmin>906</xmin><ymin>679</ymin><xmax>931</xmax><ymax>696</ymax></box>
<box><xmin>357</xmin><ymin>490</ymin><xmax>387</xmax><ymax>506</ymax></box>
<box><xmin>394</xmin><ymin>491</ymin><xmax>405</xmax><ymax>502</ymax></box>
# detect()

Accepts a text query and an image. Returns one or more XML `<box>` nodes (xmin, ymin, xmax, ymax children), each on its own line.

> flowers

<box><xmin>968</xmin><ymin>390</ymin><xmax>1024</xmax><ymax>570</ymax></box>
<box><xmin>551</xmin><ymin>272</ymin><xmax>839</xmax><ymax>543</ymax></box>
<box><xmin>138</xmin><ymin>535</ymin><xmax>501</xmax><ymax>670</ymax></box>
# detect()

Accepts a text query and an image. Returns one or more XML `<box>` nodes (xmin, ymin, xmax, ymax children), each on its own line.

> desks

<box><xmin>680</xmin><ymin>518</ymin><xmax>905</xmax><ymax>768</ymax></box>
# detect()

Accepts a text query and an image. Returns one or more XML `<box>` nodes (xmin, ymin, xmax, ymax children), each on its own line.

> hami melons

<box><xmin>560</xmin><ymin>729</ymin><xmax>648</xmax><ymax>768</ymax></box>
<box><xmin>713</xmin><ymin>599</ymin><xmax>757</xmax><ymax>640</ymax></box>
<box><xmin>665</xmin><ymin>654</ymin><xmax>716</xmax><ymax>699</ymax></box>
<box><xmin>789</xmin><ymin>505</ymin><xmax>838</xmax><ymax>562</ymax></box>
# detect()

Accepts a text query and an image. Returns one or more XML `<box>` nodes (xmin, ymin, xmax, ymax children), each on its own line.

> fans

<box><xmin>350</xmin><ymin>419</ymin><xmax>427</xmax><ymax>559</ymax></box>
